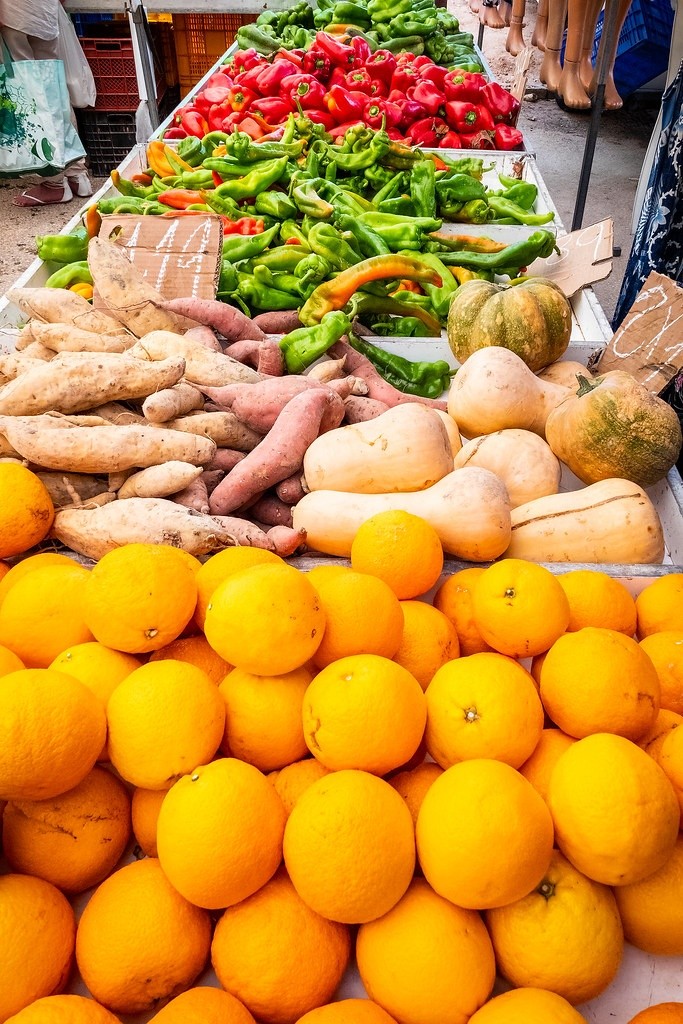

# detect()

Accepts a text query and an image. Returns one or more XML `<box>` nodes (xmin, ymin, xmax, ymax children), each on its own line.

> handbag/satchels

<box><xmin>0</xmin><ymin>34</ymin><xmax>87</xmax><ymax>173</ymax></box>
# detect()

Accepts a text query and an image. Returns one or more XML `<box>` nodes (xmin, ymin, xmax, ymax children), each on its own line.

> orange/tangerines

<box><xmin>0</xmin><ymin>461</ymin><xmax>683</xmax><ymax>1024</ymax></box>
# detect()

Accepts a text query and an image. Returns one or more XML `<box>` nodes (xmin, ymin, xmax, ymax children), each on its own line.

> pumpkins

<box><xmin>288</xmin><ymin>274</ymin><xmax>683</xmax><ymax>566</ymax></box>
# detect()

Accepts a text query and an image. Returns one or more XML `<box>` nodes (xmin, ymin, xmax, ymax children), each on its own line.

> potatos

<box><xmin>0</xmin><ymin>236</ymin><xmax>450</xmax><ymax>558</ymax></box>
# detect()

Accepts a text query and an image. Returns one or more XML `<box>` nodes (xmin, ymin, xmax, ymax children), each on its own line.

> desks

<box><xmin>0</xmin><ymin>27</ymin><xmax>683</xmax><ymax>1023</ymax></box>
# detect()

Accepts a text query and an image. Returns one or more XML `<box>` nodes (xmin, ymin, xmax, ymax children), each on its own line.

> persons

<box><xmin>0</xmin><ymin>0</ymin><xmax>93</xmax><ymax>207</ymax></box>
<box><xmin>469</xmin><ymin>0</ymin><xmax>631</xmax><ymax>108</ymax></box>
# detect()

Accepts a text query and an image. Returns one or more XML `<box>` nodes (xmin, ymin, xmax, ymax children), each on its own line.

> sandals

<box><xmin>69</xmin><ymin>173</ymin><xmax>93</xmax><ymax>197</ymax></box>
<box><xmin>13</xmin><ymin>176</ymin><xmax>73</xmax><ymax>206</ymax></box>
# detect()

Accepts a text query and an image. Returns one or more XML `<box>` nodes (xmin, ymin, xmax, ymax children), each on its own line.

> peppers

<box><xmin>34</xmin><ymin>95</ymin><xmax>561</xmax><ymax>397</ymax></box>
<box><xmin>222</xmin><ymin>0</ymin><xmax>485</xmax><ymax>72</ymax></box>
<box><xmin>162</xmin><ymin>31</ymin><xmax>525</xmax><ymax>149</ymax></box>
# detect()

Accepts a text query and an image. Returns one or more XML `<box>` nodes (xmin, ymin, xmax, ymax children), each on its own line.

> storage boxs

<box><xmin>69</xmin><ymin>13</ymin><xmax>258</xmax><ymax>177</ymax></box>
